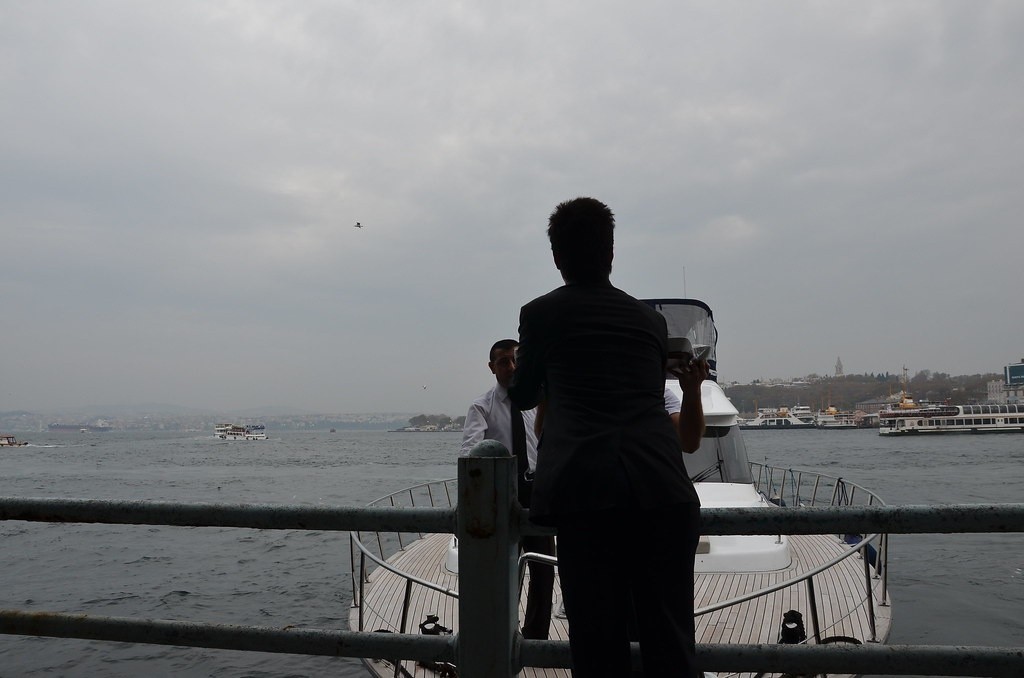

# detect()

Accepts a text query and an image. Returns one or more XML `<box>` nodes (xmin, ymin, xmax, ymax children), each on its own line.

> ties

<box><xmin>510</xmin><ymin>402</ymin><xmax>529</xmax><ymax>475</ymax></box>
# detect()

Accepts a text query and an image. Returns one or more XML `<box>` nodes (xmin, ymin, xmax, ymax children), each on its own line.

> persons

<box><xmin>508</xmin><ymin>198</ymin><xmax>711</xmax><ymax>677</ymax></box>
<box><xmin>460</xmin><ymin>339</ymin><xmax>555</xmax><ymax>640</ymax></box>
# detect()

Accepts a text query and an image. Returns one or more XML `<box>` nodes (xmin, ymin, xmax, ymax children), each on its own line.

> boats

<box><xmin>214</xmin><ymin>423</ymin><xmax>268</xmax><ymax>441</ymax></box>
<box><xmin>80</xmin><ymin>428</ymin><xmax>87</xmax><ymax>433</ymax></box>
<box><xmin>346</xmin><ymin>299</ymin><xmax>895</xmax><ymax>678</ymax></box>
<box><xmin>738</xmin><ymin>365</ymin><xmax>1024</xmax><ymax>437</ymax></box>
<box><xmin>0</xmin><ymin>435</ymin><xmax>29</xmax><ymax>447</ymax></box>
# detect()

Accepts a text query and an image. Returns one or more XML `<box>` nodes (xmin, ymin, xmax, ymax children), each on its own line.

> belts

<box><xmin>517</xmin><ymin>471</ymin><xmax>536</xmax><ymax>482</ymax></box>
